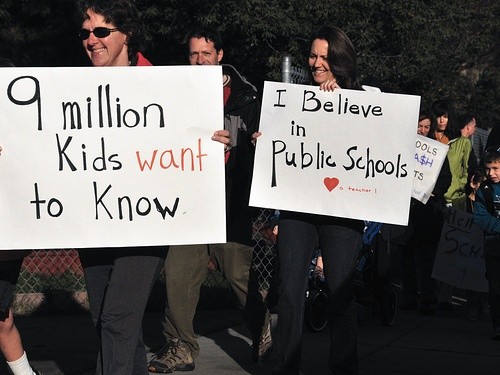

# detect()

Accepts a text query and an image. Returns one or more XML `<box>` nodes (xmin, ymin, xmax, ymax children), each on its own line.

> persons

<box><xmin>0</xmin><ymin>57</ymin><xmax>41</xmax><ymax>375</ymax></box>
<box><xmin>76</xmin><ymin>0</ymin><xmax>230</xmax><ymax>375</ymax></box>
<box><xmin>377</xmin><ymin>100</ymin><xmax>500</xmax><ymax>339</ymax></box>
<box><xmin>251</xmin><ymin>26</ymin><xmax>365</xmax><ymax>375</ymax></box>
<box><xmin>148</xmin><ymin>29</ymin><xmax>272</xmax><ymax>373</ymax></box>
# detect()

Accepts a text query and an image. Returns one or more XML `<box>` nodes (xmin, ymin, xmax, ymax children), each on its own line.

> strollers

<box><xmin>308</xmin><ymin>221</ymin><xmax>400</xmax><ymax>333</ymax></box>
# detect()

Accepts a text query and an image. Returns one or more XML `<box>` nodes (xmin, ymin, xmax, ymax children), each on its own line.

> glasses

<box><xmin>76</xmin><ymin>27</ymin><xmax>118</xmax><ymax>40</ymax></box>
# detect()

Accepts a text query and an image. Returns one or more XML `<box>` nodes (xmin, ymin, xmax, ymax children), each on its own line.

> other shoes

<box><xmin>252</xmin><ymin>316</ymin><xmax>274</xmax><ymax>367</ymax></box>
<box><xmin>30</xmin><ymin>365</ymin><xmax>42</xmax><ymax>375</ymax></box>
<box><xmin>147</xmin><ymin>340</ymin><xmax>195</xmax><ymax>374</ymax></box>
<box><xmin>466</xmin><ymin>290</ymin><xmax>492</xmax><ymax>322</ymax></box>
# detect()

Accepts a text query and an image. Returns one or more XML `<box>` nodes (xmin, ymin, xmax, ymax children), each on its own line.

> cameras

<box><xmin>472</xmin><ymin>167</ymin><xmax>486</xmax><ymax>185</ymax></box>
